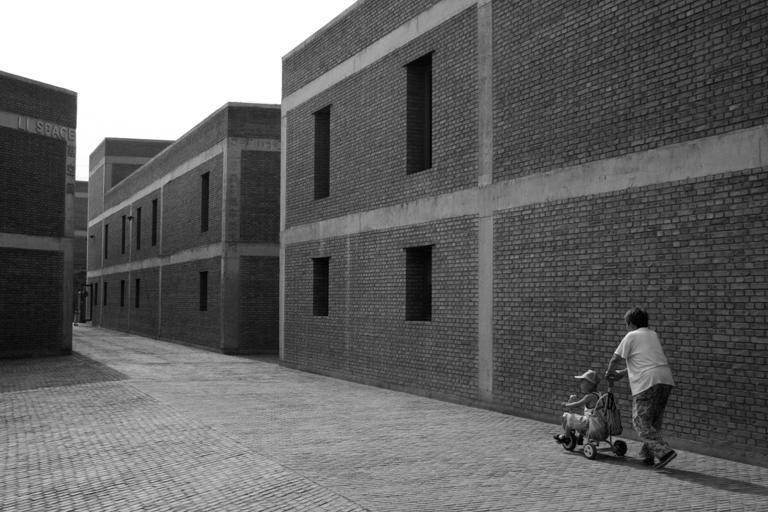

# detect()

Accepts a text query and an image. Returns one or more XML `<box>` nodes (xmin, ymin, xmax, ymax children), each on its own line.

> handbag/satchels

<box><xmin>607</xmin><ymin>408</ymin><xmax>623</xmax><ymax>435</ymax></box>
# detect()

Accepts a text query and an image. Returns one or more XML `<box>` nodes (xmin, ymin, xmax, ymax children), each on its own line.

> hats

<box><xmin>574</xmin><ymin>369</ymin><xmax>600</xmax><ymax>386</ymax></box>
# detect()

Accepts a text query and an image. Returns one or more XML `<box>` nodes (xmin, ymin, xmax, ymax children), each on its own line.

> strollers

<box><xmin>562</xmin><ymin>374</ymin><xmax>627</xmax><ymax>459</ymax></box>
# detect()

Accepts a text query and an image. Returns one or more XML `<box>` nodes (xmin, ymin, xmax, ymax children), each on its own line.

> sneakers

<box><xmin>553</xmin><ymin>433</ymin><xmax>569</xmax><ymax>441</ymax></box>
<box><xmin>654</xmin><ymin>450</ymin><xmax>678</xmax><ymax>469</ymax></box>
<box><xmin>640</xmin><ymin>457</ymin><xmax>655</xmax><ymax>465</ymax></box>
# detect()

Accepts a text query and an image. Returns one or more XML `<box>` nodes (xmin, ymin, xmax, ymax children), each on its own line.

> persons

<box><xmin>551</xmin><ymin>369</ymin><xmax>605</xmax><ymax>443</ymax></box>
<box><xmin>605</xmin><ymin>306</ymin><xmax>678</xmax><ymax>470</ymax></box>
